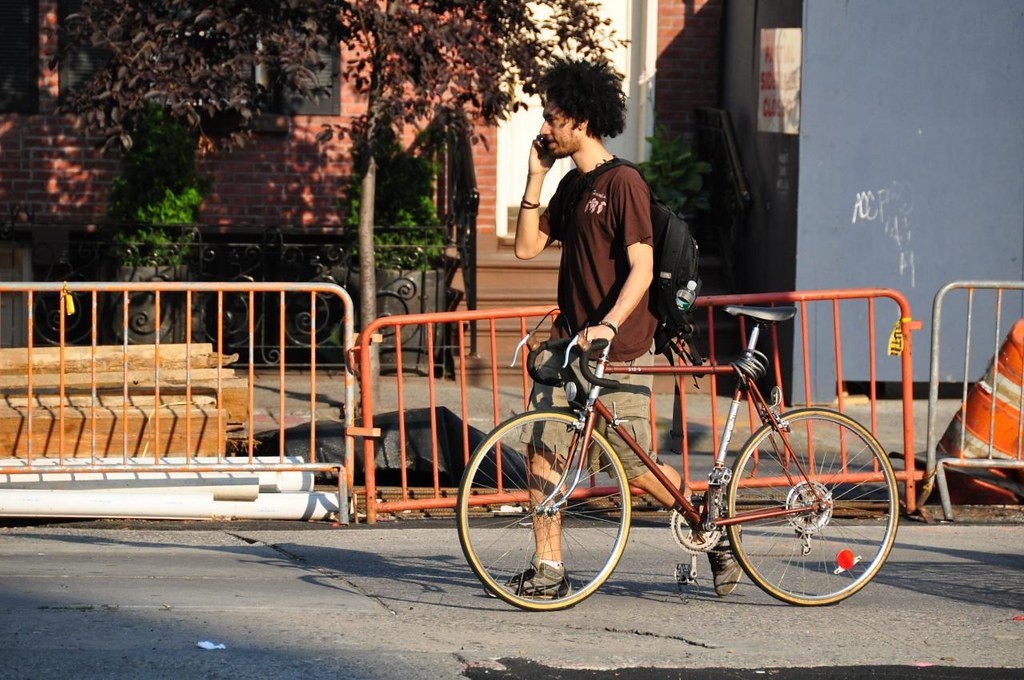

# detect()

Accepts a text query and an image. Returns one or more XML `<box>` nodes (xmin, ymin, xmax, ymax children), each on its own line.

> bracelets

<box><xmin>598</xmin><ymin>320</ymin><xmax>618</xmax><ymax>335</ymax></box>
<box><xmin>520</xmin><ymin>196</ymin><xmax>540</xmax><ymax>209</ymax></box>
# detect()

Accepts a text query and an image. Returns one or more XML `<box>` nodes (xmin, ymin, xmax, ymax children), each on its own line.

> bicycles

<box><xmin>456</xmin><ymin>304</ymin><xmax>902</xmax><ymax>608</ymax></box>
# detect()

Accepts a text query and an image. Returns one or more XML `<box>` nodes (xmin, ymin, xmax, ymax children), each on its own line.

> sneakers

<box><xmin>483</xmin><ymin>563</ymin><xmax>572</xmax><ymax>601</ymax></box>
<box><xmin>706</xmin><ymin>521</ymin><xmax>746</xmax><ymax>596</ymax></box>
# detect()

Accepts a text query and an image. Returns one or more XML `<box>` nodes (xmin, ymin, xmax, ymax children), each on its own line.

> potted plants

<box><xmin>114</xmin><ymin>96</ymin><xmax>212</xmax><ymax>343</ymax></box>
<box><xmin>330</xmin><ymin>124</ymin><xmax>446</xmax><ymax>378</ymax></box>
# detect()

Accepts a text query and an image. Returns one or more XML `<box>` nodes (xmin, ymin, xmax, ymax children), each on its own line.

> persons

<box><xmin>484</xmin><ymin>60</ymin><xmax>745</xmax><ymax>601</ymax></box>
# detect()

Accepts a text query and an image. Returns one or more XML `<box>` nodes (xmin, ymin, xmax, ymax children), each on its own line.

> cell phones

<box><xmin>541</xmin><ymin>136</ymin><xmax>550</xmax><ymax>151</ymax></box>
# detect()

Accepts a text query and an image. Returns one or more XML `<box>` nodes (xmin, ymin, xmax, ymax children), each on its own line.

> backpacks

<box><xmin>566</xmin><ymin>159</ymin><xmax>701</xmax><ymax>352</ymax></box>
